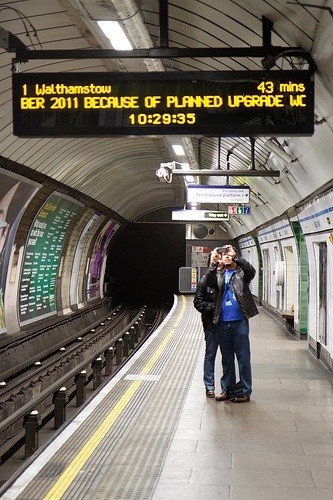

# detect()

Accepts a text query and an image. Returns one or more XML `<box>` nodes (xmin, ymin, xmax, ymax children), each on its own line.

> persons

<box><xmin>205</xmin><ymin>245</ymin><xmax>259</xmax><ymax>403</ymax></box>
<box><xmin>194</xmin><ymin>247</ymin><xmax>225</xmax><ymax>398</ymax></box>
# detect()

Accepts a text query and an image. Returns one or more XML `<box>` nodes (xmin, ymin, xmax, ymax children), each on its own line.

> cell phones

<box><xmin>216</xmin><ymin>247</ymin><xmax>228</xmax><ymax>253</ymax></box>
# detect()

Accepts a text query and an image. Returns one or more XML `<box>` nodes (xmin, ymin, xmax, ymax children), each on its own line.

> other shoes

<box><xmin>233</xmin><ymin>395</ymin><xmax>250</xmax><ymax>402</ymax></box>
<box><xmin>205</xmin><ymin>390</ymin><xmax>214</xmax><ymax>398</ymax></box>
<box><xmin>216</xmin><ymin>392</ymin><xmax>228</xmax><ymax>400</ymax></box>
